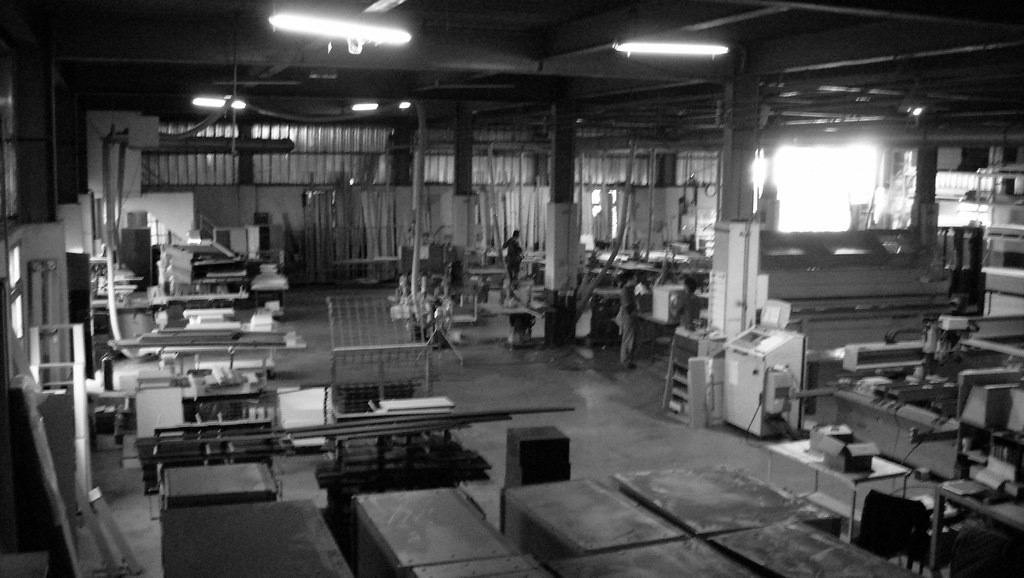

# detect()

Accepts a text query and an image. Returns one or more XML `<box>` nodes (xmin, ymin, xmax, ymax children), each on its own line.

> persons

<box><xmin>501</xmin><ymin>229</ymin><xmax>523</xmax><ymax>282</ymax></box>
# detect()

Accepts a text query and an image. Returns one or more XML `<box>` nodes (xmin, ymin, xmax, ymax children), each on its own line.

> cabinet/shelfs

<box><xmin>661</xmin><ymin>326</ymin><xmax>709</xmax><ymax>424</ymax></box>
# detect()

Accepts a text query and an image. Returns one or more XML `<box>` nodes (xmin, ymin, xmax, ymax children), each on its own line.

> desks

<box><xmin>503</xmin><ymin>478</ymin><xmax>686</xmax><ymax>564</ymax></box>
<box><xmin>610</xmin><ymin>463</ymin><xmax>841</xmax><ymax>544</ymax></box>
<box><xmin>547</xmin><ymin>537</ymin><xmax>755</xmax><ymax>578</ymax></box>
<box><xmin>763</xmin><ymin>438</ymin><xmax>908</xmax><ymax>545</ymax></box>
<box><xmin>160</xmin><ymin>499</ymin><xmax>352</xmax><ymax>578</ymax></box>
<box><xmin>407</xmin><ymin>553</ymin><xmax>552</xmax><ymax>578</ymax></box>
<box><xmin>352</xmin><ymin>488</ymin><xmax>519</xmax><ymax>578</ymax></box>
<box><xmin>708</xmin><ymin>516</ymin><xmax>921</xmax><ymax>578</ymax></box>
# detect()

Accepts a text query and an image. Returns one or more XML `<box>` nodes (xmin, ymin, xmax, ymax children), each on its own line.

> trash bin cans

<box><xmin>508</xmin><ymin>312</ymin><xmax>536</xmax><ymax>345</ymax></box>
